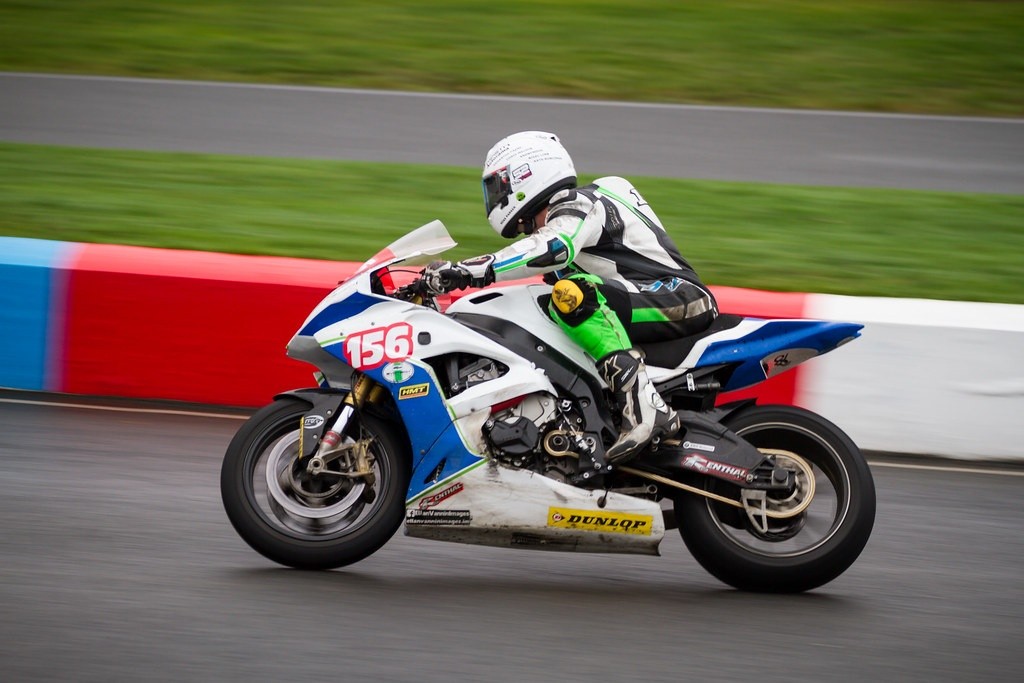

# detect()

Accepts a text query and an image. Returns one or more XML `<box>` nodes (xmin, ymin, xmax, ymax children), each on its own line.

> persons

<box><xmin>423</xmin><ymin>130</ymin><xmax>720</xmax><ymax>464</ymax></box>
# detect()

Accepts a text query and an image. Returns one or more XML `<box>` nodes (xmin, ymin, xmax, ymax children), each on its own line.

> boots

<box><xmin>601</xmin><ymin>350</ymin><xmax>679</xmax><ymax>466</ymax></box>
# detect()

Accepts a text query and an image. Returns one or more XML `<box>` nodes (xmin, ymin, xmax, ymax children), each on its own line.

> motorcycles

<box><xmin>218</xmin><ymin>217</ymin><xmax>877</xmax><ymax>594</ymax></box>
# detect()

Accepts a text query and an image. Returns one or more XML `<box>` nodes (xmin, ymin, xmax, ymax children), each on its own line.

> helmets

<box><xmin>482</xmin><ymin>129</ymin><xmax>576</xmax><ymax>238</ymax></box>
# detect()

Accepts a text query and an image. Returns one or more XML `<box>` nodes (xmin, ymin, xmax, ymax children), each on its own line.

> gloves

<box><xmin>421</xmin><ymin>259</ymin><xmax>459</xmax><ymax>295</ymax></box>
